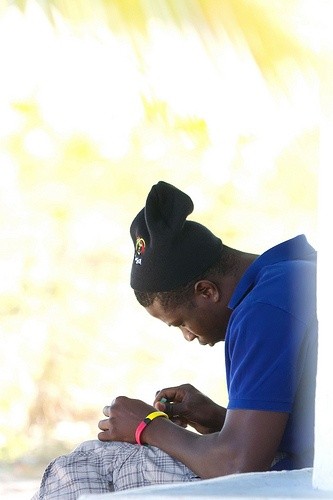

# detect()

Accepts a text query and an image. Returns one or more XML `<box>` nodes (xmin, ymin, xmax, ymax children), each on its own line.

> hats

<box><xmin>130</xmin><ymin>181</ymin><xmax>224</xmax><ymax>290</ymax></box>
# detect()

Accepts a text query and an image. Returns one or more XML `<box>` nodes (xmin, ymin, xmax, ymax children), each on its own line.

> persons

<box><xmin>29</xmin><ymin>179</ymin><xmax>320</xmax><ymax>500</ymax></box>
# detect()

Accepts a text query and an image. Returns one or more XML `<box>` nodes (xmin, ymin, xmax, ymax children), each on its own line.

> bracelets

<box><xmin>134</xmin><ymin>410</ymin><xmax>170</xmax><ymax>448</ymax></box>
